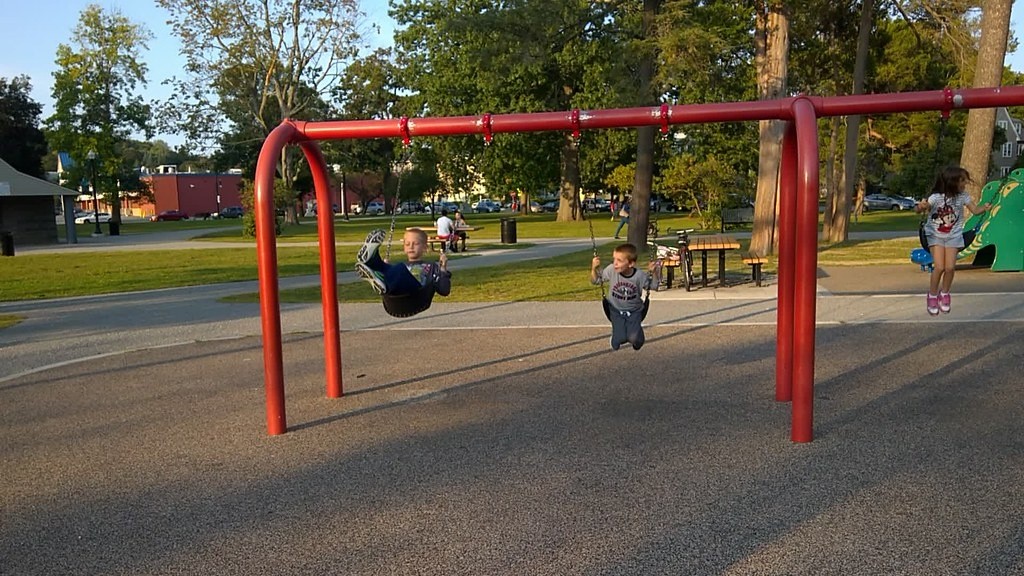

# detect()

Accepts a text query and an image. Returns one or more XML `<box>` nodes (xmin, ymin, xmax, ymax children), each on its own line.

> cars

<box><xmin>541</xmin><ymin>201</ymin><xmax>560</xmax><ymax>211</ymax></box>
<box><xmin>75</xmin><ymin>212</ymin><xmax>113</xmax><ymax>224</ymax></box>
<box><xmin>149</xmin><ymin>211</ymin><xmax>189</xmax><ymax>222</ymax></box>
<box><xmin>531</xmin><ymin>200</ymin><xmax>543</xmax><ymax>213</ymax></box>
<box><xmin>401</xmin><ymin>201</ymin><xmax>458</xmax><ymax>214</ymax></box>
<box><xmin>650</xmin><ymin>195</ymin><xmax>686</xmax><ymax>213</ymax></box>
<box><xmin>863</xmin><ymin>194</ymin><xmax>915</xmax><ymax>211</ymax></box>
<box><xmin>351</xmin><ymin>201</ymin><xmax>385</xmax><ymax>214</ymax></box>
<box><xmin>476</xmin><ymin>202</ymin><xmax>500</xmax><ymax>213</ymax></box>
<box><xmin>472</xmin><ymin>199</ymin><xmax>512</xmax><ymax>210</ymax></box>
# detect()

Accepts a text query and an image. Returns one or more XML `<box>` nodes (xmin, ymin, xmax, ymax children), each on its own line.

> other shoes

<box><xmin>615</xmin><ymin>236</ymin><xmax>620</xmax><ymax>239</ymax></box>
<box><xmin>609</xmin><ymin>336</ymin><xmax>620</xmax><ymax>351</ymax></box>
<box><xmin>610</xmin><ymin>218</ymin><xmax>615</xmax><ymax>222</ymax></box>
<box><xmin>462</xmin><ymin>247</ymin><xmax>466</xmax><ymax>252</ymax></box>
<box><xmin>449</xmin><ymin>247</ymin><xmax>456</xmax><ymax>252</ymax></box>
<box><xmin>633</xmin><ymin>339</ymin><xmax>645</xmax><ymax>351</ymax></box>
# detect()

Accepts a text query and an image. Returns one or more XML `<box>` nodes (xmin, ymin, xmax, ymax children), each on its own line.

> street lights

<box><xmin>87</xmin><ymin>149</ymin><xmax>104</xmax><ymax>238</ymax></box>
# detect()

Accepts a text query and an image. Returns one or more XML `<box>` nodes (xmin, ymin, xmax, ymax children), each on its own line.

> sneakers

<box><xmin>939</xmin><ymin>290</ymin><xmax>951</xmax><ymax>313</ymax></box>
<box><xmin>926</xmin><ymin>293</ymin><xmax>939</xmax><ymax>315</ymax></box>
<box><xmin>354</xmin><ymin>262</ymin><xmax>387</xmax><ymax>296</ymax></box>
<box><xmin>356</xmin><ymin>229</ymin><xmax>386</xmax><ymax>263</ymax></box>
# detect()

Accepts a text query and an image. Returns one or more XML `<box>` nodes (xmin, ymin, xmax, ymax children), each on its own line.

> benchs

<box><xmin>740</xmin><ymin>251</ymin><xmax>769</xmax><ymax>287</ymax></box>
<box><xmin>657</xmin><ymin>258</ymin><xmax>681</xmax><ymax>288</ymax></box>
<box><xmin>720</xmin><ymin>208</ymin><xmax>754</xmax><ymax>232</ymax></box>
<box><xmin>400</xmin><ymin>236</ymin><xmax>469</xmax><ymax>252</ymax></box>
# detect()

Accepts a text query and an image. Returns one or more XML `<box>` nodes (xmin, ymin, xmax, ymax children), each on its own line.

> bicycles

<box><xmin>676</xmin><ymin>228</ymin><xmax>696</xmax><ymax>292</ymax></box>
<box><xmin>646</xmin><ymin>241</ymin><xmax>676</xmax><ymax>291</ymax></box>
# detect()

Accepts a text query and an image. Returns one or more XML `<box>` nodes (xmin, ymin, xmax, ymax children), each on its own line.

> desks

<box><xmin>687</xmin><ymin>235</ymin><xmax>740</xmax><ymax>288</ymax></box>
<box><xmin>405</xmin><ymin>226</ymin><xmax>485</xmax><ymax>251</ymax></box>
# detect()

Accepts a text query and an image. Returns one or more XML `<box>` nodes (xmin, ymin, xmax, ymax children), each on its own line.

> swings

<box><xmin>918</xmin><ymin>113</ymin><xmax>1024</xmax><ymax>255</ymax></box>
<box><xmin>381</xmin><ymin>135</ymin><xmax>493</xmax><ymax>317</ymax></box>
<box><xmin>571</xmin><ymin>126</ymin><xmax>670</xmax><ymax>324</ymax></box>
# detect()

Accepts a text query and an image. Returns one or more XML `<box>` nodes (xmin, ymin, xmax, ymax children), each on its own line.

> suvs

<box><xmin>582</xmin><ymin>197</ymin><xmax>612</xmax><ymax>211</ymax></box>
<box><xmin>210</xmin><ymin>207</ymin><xmax>245</xmax><ymax>219</ymax></box>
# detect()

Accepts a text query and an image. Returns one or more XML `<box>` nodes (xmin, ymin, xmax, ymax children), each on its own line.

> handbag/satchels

<box><xmin>656</xmin><ymin>246</ymin><xmax>680</xmax><ymax>260</ymax></box>
<box><xmin>644</xmin><ymin>259</ymin><xmax>664</xmax><ymax>292</ymax></box>
<box><xmin>619</xmin><ymin>209</ymin><xmax>629</xmax><ymax>217</ymax></box>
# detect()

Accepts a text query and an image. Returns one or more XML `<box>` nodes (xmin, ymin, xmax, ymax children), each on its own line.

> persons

<box><xmin>354</xmin><ymin>228</ymin><xmax>452</xmax><ymax>296</ymax></box>
<box><xmin>453</xmin><ymin>212</ymin><xmax>467</xmax><ymax>252</ymax></box>
<box><xmin>436</xmin><ymin>209</ymin><xmax>459</xmax><ymax>253</ymax></box>
<box><xmin>914</xmin><ymin>166</ymin><xmax>992</xmax><ymax>316</ymax></box>
<box><xmin>590</xmin><ymin>244</ymin><xmax>660</xmax><ymax>351</ymax></box>
<box><xmin>610</xmin><ymin>196</ymin><xmax>630</xmax><ymax>239</ymax></box>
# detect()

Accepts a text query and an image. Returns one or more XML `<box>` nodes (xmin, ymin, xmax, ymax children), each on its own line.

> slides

<box><xmin>910</xmin><ymin>169</ymin><xmax>1019</xmax><ymax>267</ymax></box>
